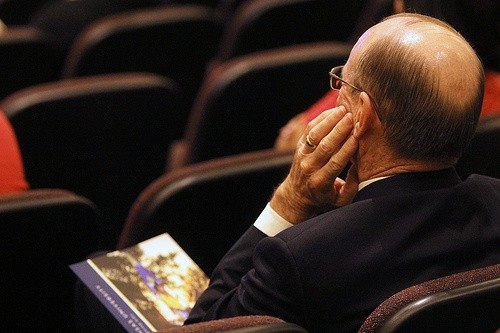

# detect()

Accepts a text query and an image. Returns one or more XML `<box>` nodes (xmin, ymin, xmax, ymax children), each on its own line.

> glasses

<box><xmin>329</xmin><ymin>66</ymin><xmax>383</xmax><ymax>122</ymax></box>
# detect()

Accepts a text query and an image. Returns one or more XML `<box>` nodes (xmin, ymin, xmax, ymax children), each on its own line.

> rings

<box><xmin>306</xmin><ymin>135</ymin><xmax>317</xmax><ymax>149</ymax></box>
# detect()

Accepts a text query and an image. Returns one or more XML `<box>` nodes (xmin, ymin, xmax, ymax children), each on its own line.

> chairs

<box><xmin>117</xmin><ymin>146</ymin><xmax>298</xmax><ymax>282</ymax></box>
<box><xmin>0</xmin><ymin>72</ymin><xmax>180</xmax><ymax>244</ymax></box>
<box><xmin>211</xmin><ymin>0</ymin><xmax>369</xmax><ymax>71</ymax></box>
<box><xmin>356</xmin><ymin>265</ymin><xmax>500</xmax><ymax>333</ymax></box>
<box><xmin>179</xmin><ymin>40</ymin><xmax>357</xmax><ymax>168</ymax></box>
<box><xmin>470</xmin><ymin>117</ymin><xmax>500</xmax><ymax>179</ymax></box>
<box><xmin>0</xmin><ymin>189</ymin><xmax>110</xmax><ymax>333</ymax></box>
<box><xmin>1</xmin><ymin>37</ymin><xmax>64</xmax><ymax>100</ymax></box>
<box><xmin>151</xmin><ymin>314</ymin><xmax>308</xmax><ymax>333</ymax></box>
<box><xmin>30</xmin><ymin>0</ymin><xmax>138</xmax><ymax>63</ymax></box>
<box><xmin>62</xmin><ymin>4</ymin><xmax>223</xmax><ymax>140</ymax></box>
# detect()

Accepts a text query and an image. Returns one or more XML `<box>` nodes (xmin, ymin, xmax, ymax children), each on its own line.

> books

<box><xmin>69</xmin><ymin>232</ymin><xmax>210</xmax><ymax>333</ymax></box>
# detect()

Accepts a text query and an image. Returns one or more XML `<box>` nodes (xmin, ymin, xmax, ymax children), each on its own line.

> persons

<box><xmin>78</xmin><ymin>13</ymin><xmax>500</xmax><ymax>333</ymax></box>
